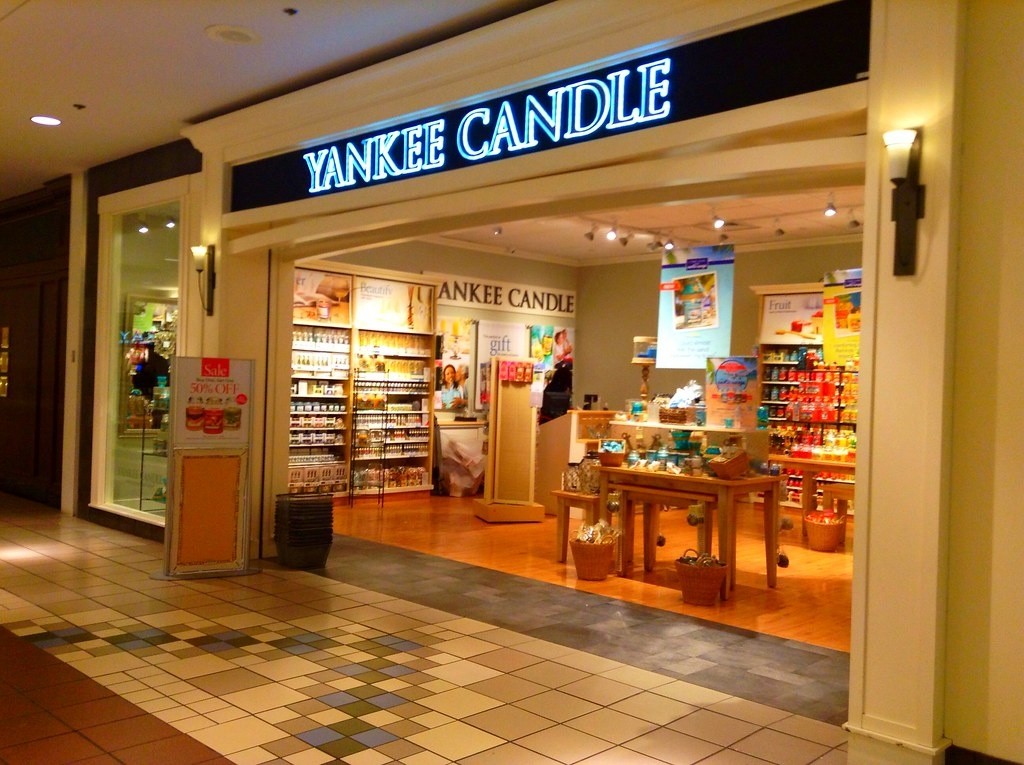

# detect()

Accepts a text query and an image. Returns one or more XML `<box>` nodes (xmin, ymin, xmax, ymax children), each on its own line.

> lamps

<box><xmin>138</xmin><ymin>225</ymin><xmax>148</xmax><ymax>234</ymax></box>
<box><xmin>627</xmin><ymin>335</ymin><xmax>656</xmax><ymax>422</ymax></box>
<box><xmin>824</xmin><ymin>193</ymin><xmax>837</xmax><ymax>217</ymax></box>
<box><xmin>607</xmin><ymin>226</ymin><xmax>618</xmax><ymax>240</ymax></box>
<box><xmin>646</xmin><ymin>231</ymin><xmax>664</xmax><ymax>252</ymax></box>
<box><xmin>663</xmin><ymin>236</ymin><xmax>675</xmax><ymax>250</ymax></box>
<box><xmin>619</xmin><ymin>232</ymin><xmax>635</xmax><ymax>246</ymax></box>
<box><xmin>190</xmin><ymin>244</ymin><xmax>215</xmax><ymax>317</ymax></box>
<box><xmin>166</xmin><ymin>218</ymin><xmax>176</xmax><ymax>228</ymax></box>
<box><xmin>712</xmin><ymin>216</ymin><xmax>725</xmax><ymax>230</ymax></box>
<box><xmin>584</xmin><ymin>222</ymin><xmax>600</xmax><ymax>241</ymax></box>
<box><xmin>882</xmin><ymin>128</ymin><xmax>928</xmax><ymax>277</ymax></box>
<box><xmin>293</xmin><ymin>300</ymin><xmax>329</xmax><ymax>320</ymax></box>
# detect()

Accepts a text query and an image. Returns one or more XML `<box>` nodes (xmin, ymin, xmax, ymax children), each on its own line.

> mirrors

<box><xmin>117</xmin><ymin>293</ymin><xmax>178</xmax><ymax>439</ymax></box>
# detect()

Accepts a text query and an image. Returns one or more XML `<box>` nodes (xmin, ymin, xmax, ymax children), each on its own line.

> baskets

<box><xmin>598</xmin><ymin>439</ymin><xmax>626</xmax><ymax>467</ymax></box>
<box><xmin>659</xmin><ymin>406</ymin><xmax>689</xmax><ymax>424</ymax></box>
<box><xmin>708</xmin><ymin>449</ymin><xmax>748</xmax><ymax>480</ymax></box>
<box><xmin>274</xmin><ymin>494</ymin><xmax>333</xmax><ymax>571</ymax></box>
<box><xmin>804</xmin><ymin>509</ymin><xmax>845</xmax><ymax>552</ymax></box>
<box><xmin>569</xmin><ymin>530</ymin><xmax>616</xmax><ymax>581</ymax></box>
<box><xmin>674</xmin><ymin>549</ymin><xmax>728</xmax><ymax>606</ymax></box>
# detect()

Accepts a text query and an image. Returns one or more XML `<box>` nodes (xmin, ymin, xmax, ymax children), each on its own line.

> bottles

<box><xmin>352</xmin><ymin>444</ymin><xmax>430</xmax><ymax>458</ymax></box>
<box><xmin>765</xmin><ymin>424</ymin><xmax>855</xmax><ymax>462</ymax></box>
<box><xmin>762</xmin><ymin>385</ymin><xmax>790</xmax><ymax>403</ymax></box>
<box><xmin>763</xmin><ymin>365</ymin><xmax>797</xmax><ymax>382</ymax></box>
<box><xmin>202</xmin><ymin>397</ymin><xmax>223</xmax><ymax>434</ymax></box>
<box><xmin>185</xmin><ymin>396</ymin><xmax>205</xmax><ymax>430</ymax></box>
<box><xmin>355</xmin><ymin>428</ymin><xmax>430</xmax><ymax>443</ymax></box>
<box><xmin>353</xmin><ymin>414</ymin><xmax>422</xmax><ymax>426</ymax></box>
<box><xmin>769</xmin><ymin>405</ymin><xmax>784</xmax><ymax>417</ymax></box>
<box><xmin>223</xmin><ymin>396</ymin><xmax>241</xmax><ymax>431</ymax></box>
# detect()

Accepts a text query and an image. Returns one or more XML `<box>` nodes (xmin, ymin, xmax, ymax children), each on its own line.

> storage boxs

<box><xmin>569</xmin><ymin>538</ymin><xmax>616</xmax><ymax>580</ymax></box>
<box><xmin>674</xmin><ymin>548</ymin><xmax>729</xmax><ymax>605</ymax></box>
<box><xmin>274</xmin><ymin>492</ymin><xmax>335</xmax><ymax>571</ymax></box>
<box><xmin>803</xmin><ymin>509</ymin><xmax>847</xmax><ymax>553</ymax></box>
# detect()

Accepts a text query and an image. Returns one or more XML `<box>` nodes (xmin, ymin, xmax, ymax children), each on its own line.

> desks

<box><xmin>606</xmin><ymin>481</ymin><xmax>749</xmax><ymax>593</ymax></box>
<box><xmin>550</xmin><ymin>490</ymin><xmax>601</xmax><ymax>563</ymax></box>
<box><xmin>767</xmin><ymin>455</ymin><xmax>855</xmax><ymax>548</ymax></box>
<box><xmin>588</xmin><ymin>459</ymin><xmax>791</xmax><ymax>601</ymax></box>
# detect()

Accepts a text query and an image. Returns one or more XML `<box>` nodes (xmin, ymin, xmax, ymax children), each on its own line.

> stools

<box><xmin>816</xmin><ymin>482</ymin><xmax>856</xmax><ymax>543</ymax></box>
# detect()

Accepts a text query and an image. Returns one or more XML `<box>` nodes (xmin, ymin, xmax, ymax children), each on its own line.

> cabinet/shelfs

<box><xmin>350</xmin><ymin>264</ymin><xmax>435</xmax><ymax>497</ymax></box>
<box><xmin>289</xmin><ymin>259</ymin><xmax>355</xmax><ymax>498</ymax></box>
<box><xmin>748</xmin><ymin>280</ymin><xmax>862</xmax><ymax>515</ymax></box>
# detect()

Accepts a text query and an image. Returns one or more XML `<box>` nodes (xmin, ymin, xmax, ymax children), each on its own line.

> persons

<box><xmin>449</xmin><ymin>365</ymin><xmax>469</xmax><ymax>409</ymax></box>
<box><xmin>131</xmin><ymin>343</ymin><xmax>169</xmax><ymax>397</ymax></box>
<box><xmin>539</xmin><ymin>368</ymin><xmax>572</xmax><ymax>425</ymax></box>
<box><xmin>531</xmin><ymin>325</ymin><xmax>573</xmax><ymax>372</ymax></box>
<box><xmin>441</xmin><ymin>365</ymin><xmax>461</xmax><ymax>409</ymax></box>
